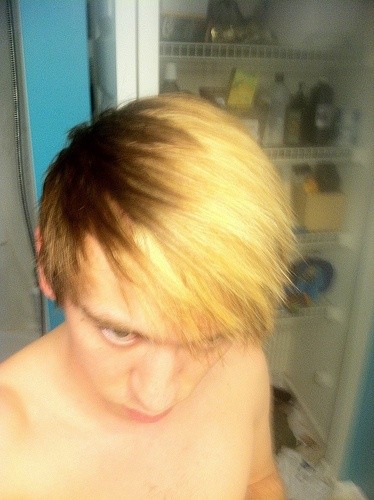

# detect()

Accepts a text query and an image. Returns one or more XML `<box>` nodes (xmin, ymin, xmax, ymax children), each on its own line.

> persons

<box><xmin>0</xmin><ymin>93</ymin><xmax>305</xmax><ymax>500</ymax></box>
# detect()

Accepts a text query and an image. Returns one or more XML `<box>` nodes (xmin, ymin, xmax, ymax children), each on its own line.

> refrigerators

<box><xmin>13</xmin><ymin>0</ymin><xmax>374</xmax><ymax>500</ymax></box>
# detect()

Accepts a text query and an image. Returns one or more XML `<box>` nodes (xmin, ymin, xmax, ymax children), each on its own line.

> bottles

<box><xmin>286</xmin><ymin>80</ymin><xmax>309</xmax><ymax>147</ymax></box>
<box><xmin>158</xmin><ymin>60</ymin><xmax>180</xmax><ymax>94</ymax></box>
<box><xmin>304</xmin><ymin>74</ymin><xmax>335</xmax><ymax>145</ymax></box>
<box><xmin>265</xmin><ymin>70</ymin><xmax>291</xmax><ymax>145</ymax></box>
